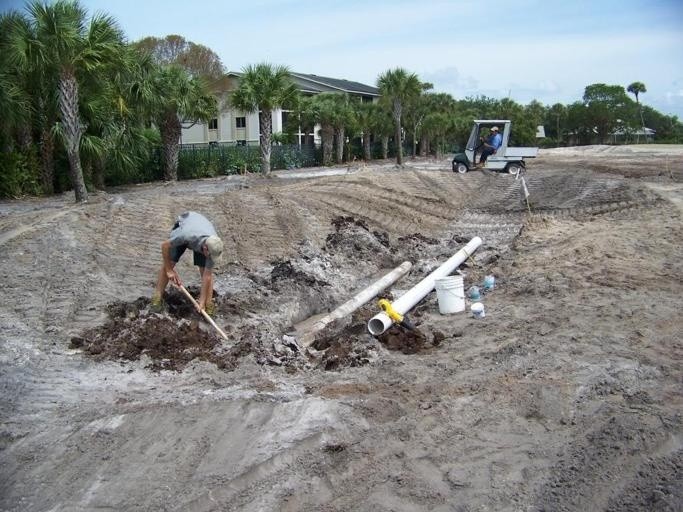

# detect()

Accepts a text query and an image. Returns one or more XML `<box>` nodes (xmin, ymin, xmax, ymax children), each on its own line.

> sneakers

<box><xmin>475</xmin><ymin>163</ymin><xmax>485</xmax><ymax>168</ymax></box>
<box><xmin>151</xmin><ymin>286</ymin><xmax>162</xmax><ymax>312</ymax></box>
<box><xmin>204</xmin><ymin>302</ymin><xmax>215</xmax><ymax>323</ymax></box>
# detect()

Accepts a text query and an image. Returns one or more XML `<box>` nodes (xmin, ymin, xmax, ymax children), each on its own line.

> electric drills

<box><xmin>377</xmin><ymin>298</ymin><xmax>436</xmax><ymax>349</ymax></box>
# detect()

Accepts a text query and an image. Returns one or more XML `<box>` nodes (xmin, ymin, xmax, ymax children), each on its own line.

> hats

<box><xmin>205</xmin><ymin>235</ymin><xmax>223</xmax><ymax>264</ymax></box>
<box><xmin>490</xmin><ymin>127</ymin><xmax>498</xmax><ymax>131</ymax></box>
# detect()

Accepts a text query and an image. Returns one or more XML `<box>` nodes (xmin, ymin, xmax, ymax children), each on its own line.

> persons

<box><xmin>475</xmin><ymin>125</ymin><xmax>503</xmax><ymax>170</ymax></box>
<box><xmin>150</xmin><ymin>211</ymin><xmax>223</xmax><ymax>316</ymax></box>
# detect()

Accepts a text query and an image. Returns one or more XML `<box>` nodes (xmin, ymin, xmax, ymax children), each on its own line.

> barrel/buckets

<box><xmin>433</xmin><ymin>275</ymin><xmax>465</xmax><ymax>315</ymax></box>
